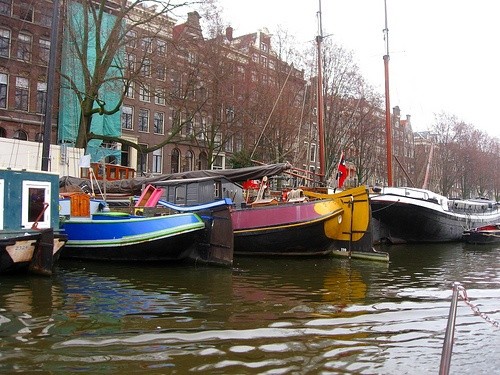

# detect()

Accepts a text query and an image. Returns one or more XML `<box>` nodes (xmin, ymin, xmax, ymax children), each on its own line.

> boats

<box><xmin>1</xmin><ymin>1</ymin><xmax>497</xmax><ymax>268</ymax></box>
